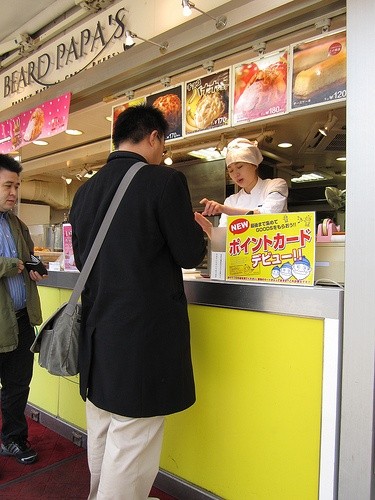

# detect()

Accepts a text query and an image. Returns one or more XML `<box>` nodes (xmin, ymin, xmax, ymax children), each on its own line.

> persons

<box><xmin>71</xmin><ymin>105</ymin><xmax>205</xmax><ymax>500</ymax></box>
<box><xmin>194</xmin><ymin>138</ymin><xmax>288</xmax><ymax>240</ymax></box>
<box><xmin>0</xmin><ymin>151</ymin><xmax>49</xmax><ymax>465</ymax></box>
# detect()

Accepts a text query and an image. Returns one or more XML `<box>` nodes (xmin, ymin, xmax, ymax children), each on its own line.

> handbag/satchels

<box><xmin>30</xmin><ymin>303</ymin><xmax>84</xmax><ymax>377</ymax></box>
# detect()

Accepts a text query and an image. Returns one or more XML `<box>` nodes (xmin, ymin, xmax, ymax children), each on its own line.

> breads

<box><xmin>23</xmin><ymin>108</ymin><xmax>44</xmax><ymax>141</ymax></box>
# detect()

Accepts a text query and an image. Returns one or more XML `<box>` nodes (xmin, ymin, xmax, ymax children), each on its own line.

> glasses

<box><xmin>156</xmin><ymin>134</ymin><xmax>167</xmax><ymax>156</ymax></box>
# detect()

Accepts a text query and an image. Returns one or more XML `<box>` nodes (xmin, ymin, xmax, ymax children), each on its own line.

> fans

<box><xmin>325</xmin><ymin>186</ymin><xmax>346</xmax><ymax>210</ymax></box>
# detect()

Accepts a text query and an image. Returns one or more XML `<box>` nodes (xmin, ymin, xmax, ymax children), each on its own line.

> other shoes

<box><xmin>1</xmin><ymin>437</ymin><xmax>38</xmax><ymax>464</ymax></box>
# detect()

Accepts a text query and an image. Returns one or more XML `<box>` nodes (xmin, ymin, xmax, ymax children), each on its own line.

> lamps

<box><xmin>181</xmin><ymin>0</ymin><xmax>227</xmax><ymax>29</ymax></box>
<box><xmin>123</xmin><ymin>30</ymin><xmax>169</xmax><ymax>54</ymax></box>
<box><xmin>61</xmin><ymin>109</ymin><xmax>338</xmax><ymax>184</ymax></box>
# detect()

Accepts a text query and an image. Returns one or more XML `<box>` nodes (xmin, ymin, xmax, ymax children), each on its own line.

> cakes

<box><xmin>152</xmin><ymin>94</ymin><xmax>181</xmax><ymax>133</ymax></box>
<box><xmin>294</xmin><ymin>51</ymin><xmax>346</xmax><ymax>96</ymax></box>
